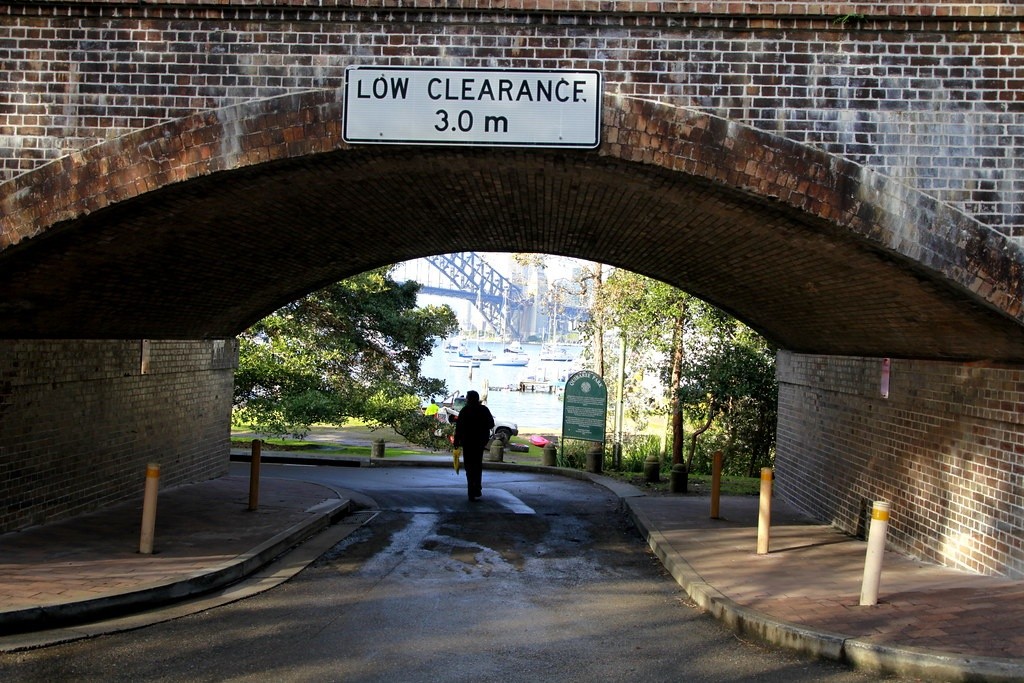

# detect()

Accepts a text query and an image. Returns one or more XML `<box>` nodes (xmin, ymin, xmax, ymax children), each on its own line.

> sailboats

<box><xmin>445</xmin><ymin>285</ymin><xmax>573</xmax><ymax>367</ymax></box>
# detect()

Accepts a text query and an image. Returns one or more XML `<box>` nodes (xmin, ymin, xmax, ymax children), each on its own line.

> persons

<box><xmin>455</xmin><ymin>391</ymin><xmax>495</xmax><ymax>502</ymax></box>
<box><xmin>425</xmin><ymin>398</ymin><xmax>439</xmax><ymax>416</ymax></box>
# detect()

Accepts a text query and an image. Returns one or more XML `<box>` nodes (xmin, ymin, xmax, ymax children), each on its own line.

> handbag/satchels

<box><xmin>453</xmin><ymin>448</ymin><xmax>462</xmax><ymax>475</ymax></box>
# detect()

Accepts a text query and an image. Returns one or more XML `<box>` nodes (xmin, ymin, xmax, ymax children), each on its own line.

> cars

<box><xmin>434</xmin><ymin>408</ymin><xmax>518</xmax><ymax>445</ymax></box>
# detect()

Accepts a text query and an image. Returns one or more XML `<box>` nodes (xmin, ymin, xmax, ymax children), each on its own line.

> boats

<box><xmin>529</xmin><ymin>434</ymin><xmax>549</xmax><ymax>447</ymax></box>
